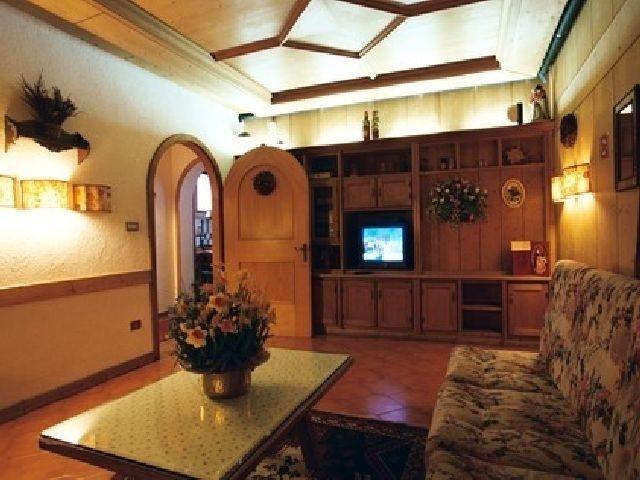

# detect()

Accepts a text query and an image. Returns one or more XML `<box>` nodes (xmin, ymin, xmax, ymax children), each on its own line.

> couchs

<box><xmin>423</xmin><ymin>257</ymin><xmax>639</xmax><ymax>479</ymax></box>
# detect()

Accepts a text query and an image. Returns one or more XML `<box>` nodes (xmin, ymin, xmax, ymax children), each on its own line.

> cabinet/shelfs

<box><xmin>341</xmin><ymin>141</ymin><xmax>419</xmax><ymax>340</ymax></box>
<box><xmin>291</xmin><ymin>144</ymin><xmax>341</xmax><ymax>335</ymax></box>
<box><xmin>416</xmin><ymin>121</ymin><xmax>552</xmax><ymax>351</ymax></box>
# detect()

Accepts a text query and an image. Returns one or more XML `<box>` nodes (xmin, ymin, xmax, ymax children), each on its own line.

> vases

<box><xmin>203</xmin><ymin>370</ymin><xmax>251</xmax><ymax>398</ymax></box>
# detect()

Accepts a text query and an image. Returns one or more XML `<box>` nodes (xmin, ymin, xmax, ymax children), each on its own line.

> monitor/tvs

<box><xmin>357</xmin><ymin>224</ymin><xmax>410</xmax><ymax>269</ymax></box>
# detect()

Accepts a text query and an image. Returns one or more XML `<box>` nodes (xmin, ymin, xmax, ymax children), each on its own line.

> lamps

<box><xmin>1</xmin><ymin>175</ymin><xmax>111</xmax><ymax>215</ymax></box>
<box><xmin>550</xmin><ymin>163</ymin><xmax>590</xmax><ymax>204</ymax></box>
<box><xmin>276</xmin><ymin>0</ymin><xmax>410</xmax><ymax>60</ymax></box>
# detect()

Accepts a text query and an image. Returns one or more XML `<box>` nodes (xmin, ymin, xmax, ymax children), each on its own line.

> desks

<box><xmin>39</xmin><ymin>345</ymin><xmax>354</xmax><ymax>480</ymax></box>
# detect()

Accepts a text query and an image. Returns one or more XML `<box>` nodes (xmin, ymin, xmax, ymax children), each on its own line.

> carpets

<box><xmin>110</xmin><ymin>408</ymin><xmax>429</xmax><ymax>480</ymax></box>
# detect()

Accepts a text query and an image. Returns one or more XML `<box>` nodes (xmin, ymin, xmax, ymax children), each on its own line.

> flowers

<box><xmin>166</xmin><ymin>262</ymin><xmax>276</xmax><ymax>367</ymax></box>
<box><xmin>427</xmin><ymin>175</ymin><xmax>489</xmax><ymax>232</ymax></box>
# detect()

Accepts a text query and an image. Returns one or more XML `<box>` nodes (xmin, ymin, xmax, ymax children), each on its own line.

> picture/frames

<box><xmin>612</xmin><ymin>83</ymin><xmax>638</xmax><ymax>194</ymax></box>
